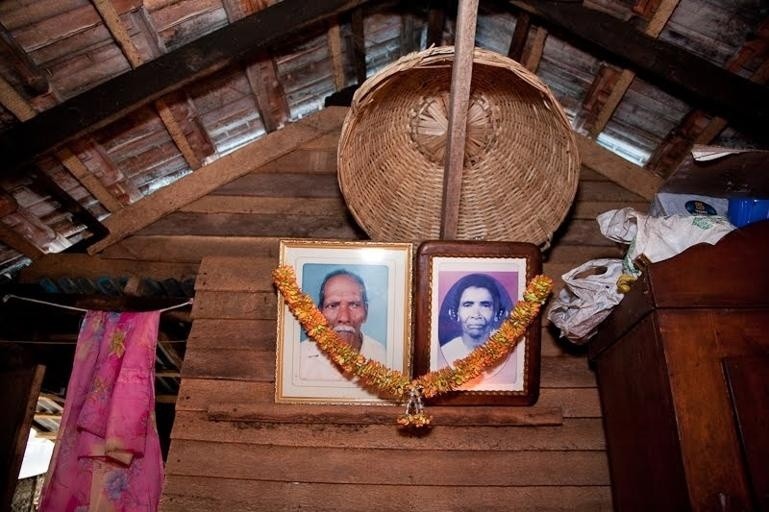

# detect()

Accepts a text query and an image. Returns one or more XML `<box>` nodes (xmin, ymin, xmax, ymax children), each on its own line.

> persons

<box><xmin>441</xmin><ymin>275</ymin><xmax>513</xmax><ymax>386</ymax></box>
<box><xmin>300</xmin><ymin>267</ymin><xmax>384</xmax><ymax>384</ymax></box>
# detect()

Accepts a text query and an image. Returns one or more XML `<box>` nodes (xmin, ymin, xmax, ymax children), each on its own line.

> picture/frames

<box><xmin>273</xmin><ymin>238</ymin><xmax>413</xmax><ymax>407</ymax></box>
<box><xmin>416</xmin><ymin>239</ymin><xmax>540</xmax><ymax>407</ymax></box>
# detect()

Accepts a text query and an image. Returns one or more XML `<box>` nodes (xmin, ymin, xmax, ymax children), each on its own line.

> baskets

<box><xmin>335</xmin><ymin>44</ymin><xmax>583</xmax><ymax>252</ymax></box>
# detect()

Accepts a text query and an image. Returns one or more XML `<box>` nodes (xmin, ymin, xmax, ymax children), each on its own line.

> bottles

<box><xmin>631</xmin><ymin>254</ymin><xmax>653</xmax><ymax>274</ymax></box>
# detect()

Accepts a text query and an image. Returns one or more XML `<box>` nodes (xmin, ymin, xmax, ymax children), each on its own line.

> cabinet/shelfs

<box><xmin>586</xmin><ymin>219</ymin><xmax>767</xmax><ymax>512</ymax></box>
